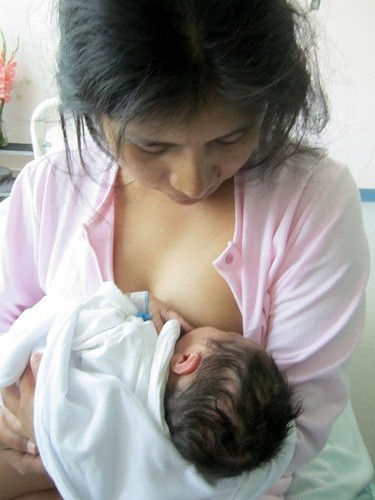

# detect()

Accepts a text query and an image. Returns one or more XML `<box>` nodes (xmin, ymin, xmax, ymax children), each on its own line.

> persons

<box><xmin>0</xmin><ymin>290</ymin><xmax>302</xmax><ymax>476</ymax></box>
<box><xmin>0</xmin><ymin>0</ymin><xmax>372</xmax><ymax>500</ymax></box>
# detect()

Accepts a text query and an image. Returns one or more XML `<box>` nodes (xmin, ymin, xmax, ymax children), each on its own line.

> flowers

<box><xmin>0</xmin><ymin>29</ymin><xmax>21</xmax><ymax>102</ymax></box>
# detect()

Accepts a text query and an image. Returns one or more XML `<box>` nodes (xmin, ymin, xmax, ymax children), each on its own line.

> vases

<box><xmin>0</xmin><ymin>97</ymin><xmax>10</xmax><ymax>146</ymax></box>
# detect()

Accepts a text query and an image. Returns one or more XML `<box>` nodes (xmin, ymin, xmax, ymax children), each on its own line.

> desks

<box><xmin>0</xmin><ymin>143</ymin><xmax>34</xmax><ymax>156</ymax></box>
